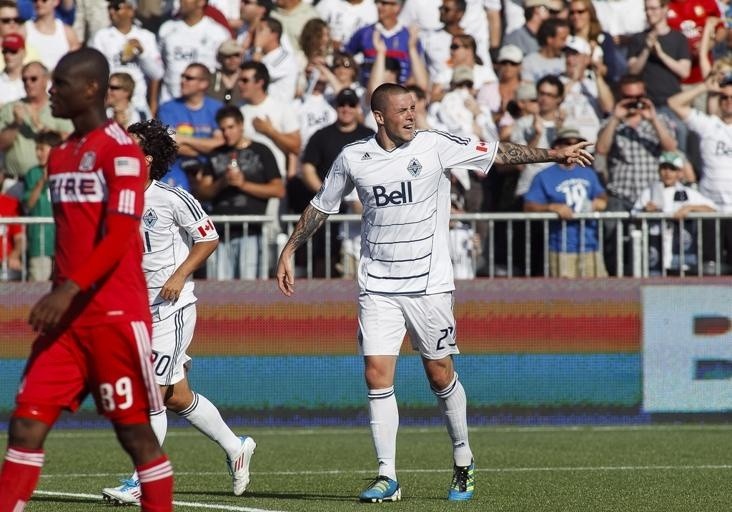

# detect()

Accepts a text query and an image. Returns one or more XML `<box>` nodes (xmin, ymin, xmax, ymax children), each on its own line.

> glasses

<box><xmin>2</xmin><ymin>4</ymin><xmax>256</xmax><ymax>91</ymax></box>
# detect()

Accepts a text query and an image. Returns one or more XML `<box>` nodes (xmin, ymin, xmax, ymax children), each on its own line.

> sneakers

<box><xmin>358</xmin><ymin>475</ymin><xmax>402</xmax><ymax>503</ymax></box>
<box><xmin>102</xmin><ymin>478</ymin><xmax>139</xmax><ymax>507</ymax></box>
<box><xmin>448</xmin><ymin>460</ymin><xmax>475</xmax><ymax>502</ymax></box>
<box><xmin>227</xmin><ymin>436</ymin><xmax>256</xmax><ymax>497</ymax></box>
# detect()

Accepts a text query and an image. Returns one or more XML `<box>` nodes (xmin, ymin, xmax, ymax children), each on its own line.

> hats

<box><xmin>551</xmin><ymin>127</ymin><xmax>587</xmax><ymax>144</ymax></box>
<box><xmin>658</xmin><ymin>151</ymin><xmax>684</xmax><ymax>171</ymax></box>
<box><xmin>453</xmin><ymin>0</ymin><xmax>593</xmax><ymax>90</ymax></box>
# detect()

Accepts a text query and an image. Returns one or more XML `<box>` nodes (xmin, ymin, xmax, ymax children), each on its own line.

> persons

<box><xmin>0</xmin><ymin>2</ymin><xmax>732</xmax><ymax>281</ymax></box>
<box><xmin>0</xmin><ymin>47</ymin><xmax>174</xmax><ymax>511</ymax></box>
<box><xmin>103</xmin><ymin>118</ymin><xmax>255</xmax><ymax>507</ymax></box>
<box><xmin>274</xmin><ymin>80</ymin><xmax>598</xmax><ymax>503</ymax></box>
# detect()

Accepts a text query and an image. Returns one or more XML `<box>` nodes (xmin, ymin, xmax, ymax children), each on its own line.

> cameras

<box><xmin>328</xmin><ymin>54</ymin><xmax>352</xmax><ymax>69</ymax></box>
<box><xmin>719</xmin><ymin>74</ymin><xmax>732</xmax><ymax>86</ymax></box>
<box><xmin>627</xmin><ymin>99</ymin><xmax>645</xmax><ymax>109</ymax></box>
